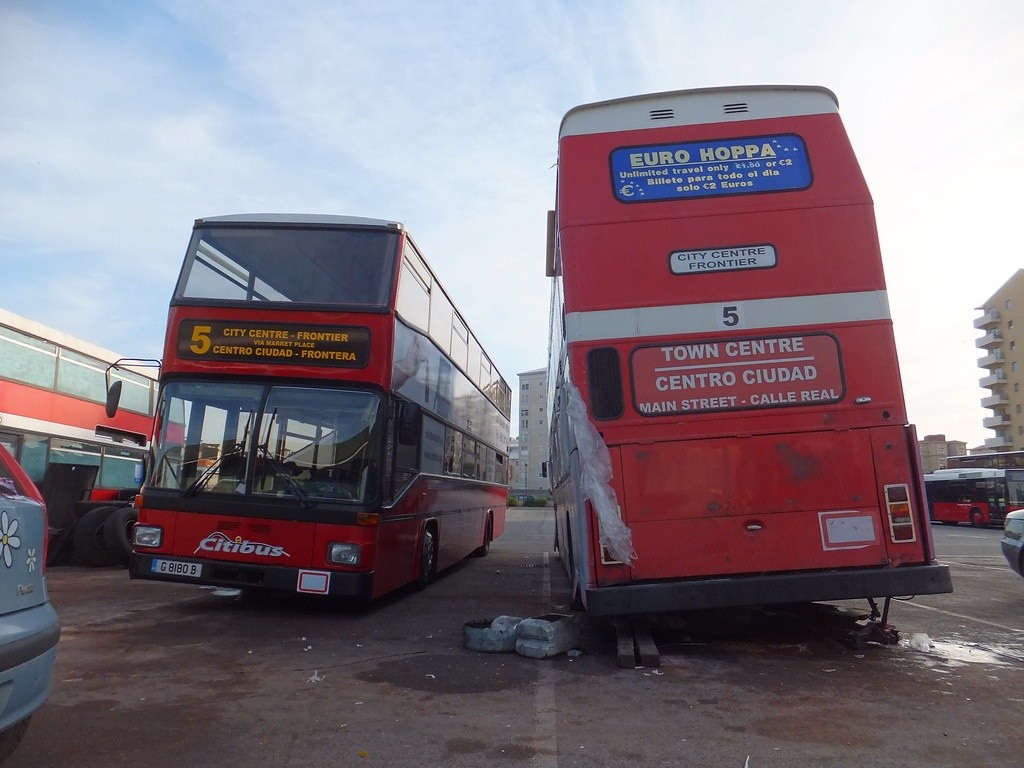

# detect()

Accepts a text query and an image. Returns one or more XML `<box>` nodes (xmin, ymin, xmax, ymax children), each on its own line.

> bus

<box><xmin>544</xmin><ymin>85</ymin><xmax>955</xmax><ymax>619</ymax></box>
<box><xmin>104</xmin><ymin>212</ymin><xmax>512</xmax><ymax>604</ymax></box>
<box><xmin>0</xmin><ymin>308</ymin><xmax>159</xmax><ymax>561</ymax></box>
<box><xmin>923</xmin><ymin>469</ymin><xmax>1024</xmax><ymax>529</ymax></box>
<box><xmin>947</xmin><ymin>450</ymin><xmax>1024</xmax><ymax>513</ymax></box>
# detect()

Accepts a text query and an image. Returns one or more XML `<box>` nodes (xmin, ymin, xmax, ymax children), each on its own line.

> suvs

<box><xmin>0</xmin><ymin>444</ymin><xmax>60</xmax><ymax>764</ymax></box>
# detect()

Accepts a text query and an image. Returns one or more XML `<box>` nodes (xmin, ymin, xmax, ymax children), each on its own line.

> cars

<box><xmin>1000</xmin><ymin>508</ymin><xmax>1024</xmax><ymax>577</ymax></box>
